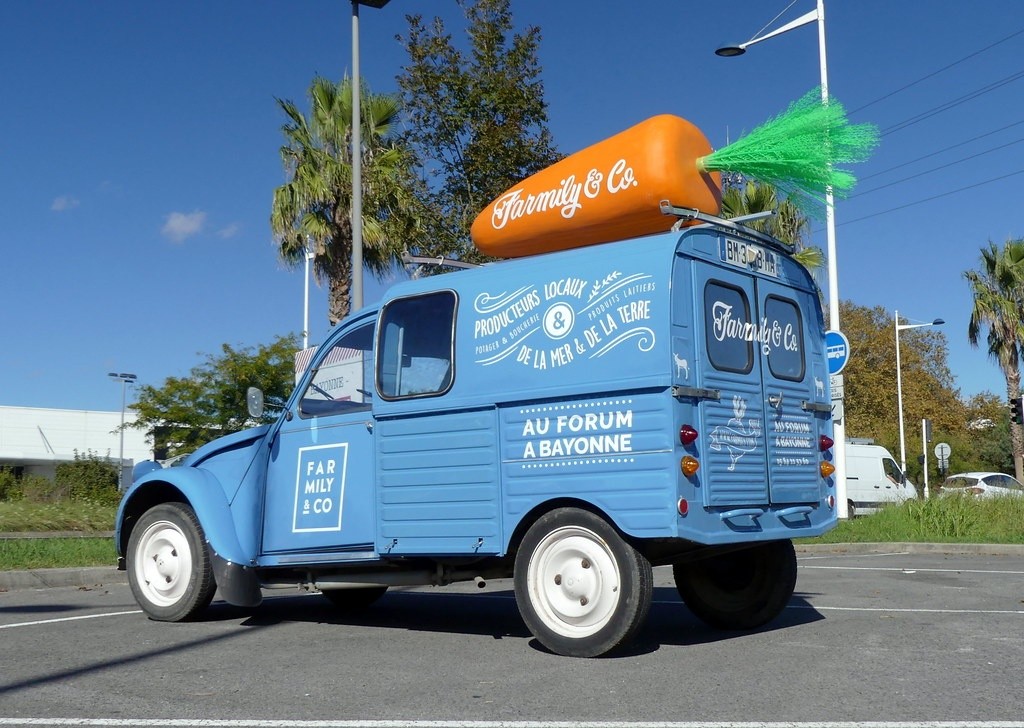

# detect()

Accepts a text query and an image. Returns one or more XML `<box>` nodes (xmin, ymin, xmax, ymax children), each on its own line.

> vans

<box><xmin>111</xmin><ymin>197</ymin><xmax>838</xmax><ymax>657</ymax></box>
<box><xmin>845</xmin><ymin>444</ymin><xmax>919</xmax><ymax>521</ymax></box>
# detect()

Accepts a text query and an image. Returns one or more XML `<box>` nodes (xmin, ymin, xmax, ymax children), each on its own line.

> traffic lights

<box><xmin>1010</xmin><ymin>398</ymin><xmax>1024</xmax><ymax>424</ymax></box>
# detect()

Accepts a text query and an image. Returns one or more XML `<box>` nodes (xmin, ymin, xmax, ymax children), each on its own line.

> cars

<box><xmin>938</xmin><ymin>471</ymin><xmax>1024</xmax><ymax>501</ymax></box>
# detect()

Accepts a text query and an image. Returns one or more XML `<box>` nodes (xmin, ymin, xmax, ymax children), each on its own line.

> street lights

<box><xmin>108</xmin><ymin>370</ymin><xmax>137</xmax><ymax>493</ymax></box>
<box><xmin>714</xmin><ymin>0</ymin><xmax>849</xmax><ymax>530</ymax></box>
<box><xmin>894</xmin><ymin>309</ymin><xmax>945</xmax><ymax>473</ymax></box>
<box><xmin>350</xmin><ymin>0</ymin><xmax>389</xmax><ymax>309</ymax></box>
